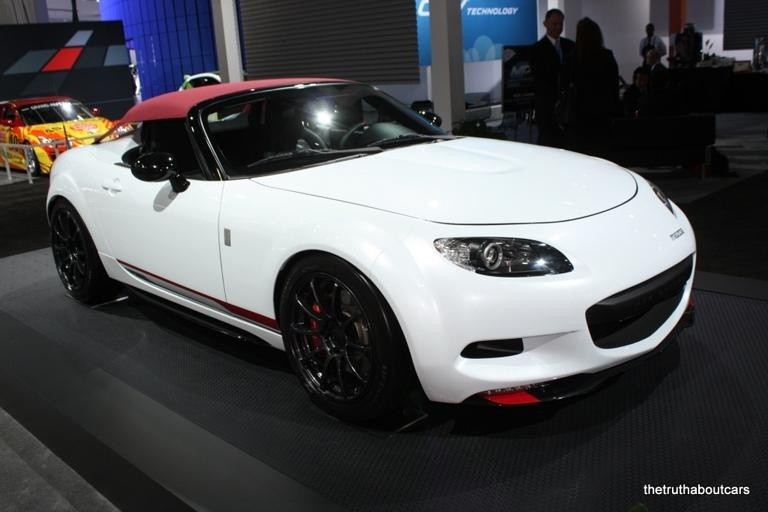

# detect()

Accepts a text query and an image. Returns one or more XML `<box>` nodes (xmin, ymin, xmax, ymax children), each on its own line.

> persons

<box><xmin>617</xmin><ymin>22</ymin><xmax>701</xmax><ymax>118</ymax></box>
<box><xmin>530</xmin><ymin>9</ymin><xmax>576</xmax><ymax>145</ymax></box>
<box><xmin>564</xmin><ymin>19</ymin><xmax>619</xmax><ymax>163</ymax></box>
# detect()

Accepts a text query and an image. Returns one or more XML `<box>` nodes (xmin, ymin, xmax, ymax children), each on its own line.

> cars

<box><xmin>178</xmin><ymin>71</ymin><xmax>221</xmax><ymax>92</ymax></box>
<box><xmin>0</xmin><ymin>95</ymin><xmax>119</xmax><ymax>177</ymax></box>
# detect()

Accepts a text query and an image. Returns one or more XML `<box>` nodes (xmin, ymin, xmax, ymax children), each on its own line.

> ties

<box><xmin>555</xmin><ymin>39</ymin><xmax>560</xmax><ymax>55</ymax></box>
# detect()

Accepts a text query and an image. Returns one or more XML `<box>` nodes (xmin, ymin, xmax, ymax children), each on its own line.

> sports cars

<box><xmin>45</xmin><ymin>76</ymin><xmax>698</xmax><ymax>425</ymax></box>
